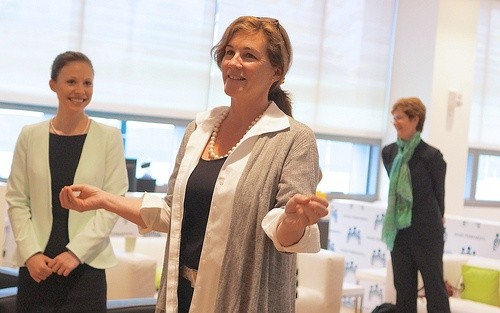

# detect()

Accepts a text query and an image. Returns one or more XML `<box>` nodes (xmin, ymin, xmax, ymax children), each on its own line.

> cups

<box><xmin>125</xmin><ymin>158</ymin><xmax>137</xmax><ymax>192</ymax></box>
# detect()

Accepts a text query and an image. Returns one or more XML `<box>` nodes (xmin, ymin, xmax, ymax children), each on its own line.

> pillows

<box><xmin>459</xmin><ymin>264</ymin><xmax>500</xmax><ymax>307</ymax></box>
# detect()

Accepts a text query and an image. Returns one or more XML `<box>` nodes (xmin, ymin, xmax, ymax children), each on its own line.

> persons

<box><xmin>59</xmin><ymin>16</ymin><xmax>331</xmax><ymax>313</ymax></box>
<box><xmin>6</xmin><ymin>52</ymin><xmax>128</xmax><ymax>313</ymax></box>
<box><xmin>381</xmin><ymin>96</ymin><xmax>451</xmax><ymax>313</ymax></box>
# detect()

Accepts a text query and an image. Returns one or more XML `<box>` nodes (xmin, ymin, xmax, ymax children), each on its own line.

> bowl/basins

<box><xmin>137</xmin><ymin>179</ymin><xmax>156</xmax><ymax>192</ymax></box>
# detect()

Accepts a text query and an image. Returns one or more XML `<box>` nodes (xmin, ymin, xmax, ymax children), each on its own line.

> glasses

<box><xmin>256</xmin><ymin>16</ymin><xmax>279</xmax><ymax>33</ymax></box>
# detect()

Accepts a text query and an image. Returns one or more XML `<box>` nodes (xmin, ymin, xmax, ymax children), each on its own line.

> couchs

<box><xmin>0</xmin><ymin>191</ymin><xmax>345</xmax><ymax>313</ymax></box>
<box><xmin>381</xmin><ymin>253</ymin><xmax>500</xmax><ymax>313</ymax></box>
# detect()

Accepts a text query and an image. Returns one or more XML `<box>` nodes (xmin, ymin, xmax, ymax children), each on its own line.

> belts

<box><xmin>178</xmin><ymin>264</ymin><xmax>198</xmax><ymax>289</ymax></box>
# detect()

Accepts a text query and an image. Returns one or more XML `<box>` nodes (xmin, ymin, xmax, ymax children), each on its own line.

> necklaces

<box><xmin>51</xmin><ymin>114</ymin><xmax>89</xmax><ymax>135</ymax></box>
<box><xmin>208</xmin><ymin>106</ymin><xmax>264</xmax><ymax>161</ymax></box>
